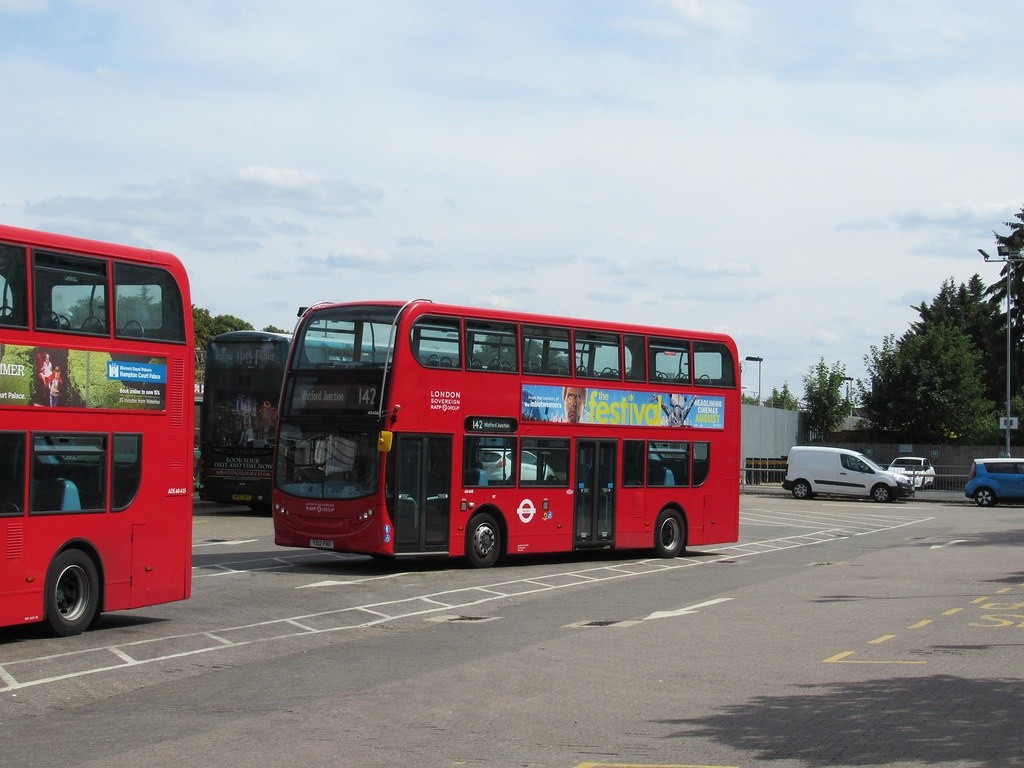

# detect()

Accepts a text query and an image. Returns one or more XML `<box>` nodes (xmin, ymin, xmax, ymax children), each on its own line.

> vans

<box><xmin>780</xmin><ymin>445</ymin><xmax>918</xmax><ymax>504</ymax></box>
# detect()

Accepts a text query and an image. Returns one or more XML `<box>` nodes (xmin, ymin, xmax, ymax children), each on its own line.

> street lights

<box><xmin>840</xmin><ymin>376</ymin><xmax>854</xmax><ymax>433</ymax></box>
<box><xmin>977</xmin><ymin>245</ymin><xmax>1024</xmax><ymax>457</ymax></box>
<box><xmin>744</xmin><ymin>355</ymin><xmax>763</xmax><ymax>407</ymax></box>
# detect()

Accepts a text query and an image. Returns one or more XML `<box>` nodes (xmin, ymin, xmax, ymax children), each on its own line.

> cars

<box><xmin>964</xmin><ymin>458</ymin><xmax>1024</xmax><ymax>506</ymax></box>
<box><xmin>479</xmin><ymin>446</ymin><xmax>556</xmax><ymax>488</ymax></box>
<box><xmin>886</xmin><ymin>457</ymin><xmax>936</xmax><ymax>489</ymax></box>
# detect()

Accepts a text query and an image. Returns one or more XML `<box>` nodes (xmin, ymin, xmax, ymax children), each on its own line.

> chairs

<box><xmin>426</xmin><ymin>354</ymin><xmax>711</xmax><ymax>385</ymax></box>
<box><xmin>40</xmin><ymin>478</ymin><xmax>81</xmax><ymax>511</ymax></box>
<box><xmin>0</xmin><ymin>305</ymin><xmax>145</xmax><ymax>337</ymax></box>
<box><xmin>465</xmin><ymin>468</ymin><xmax>488</xmax><ymax>486</ymax></box>
<box><xmin>650</xmin><ymin>467</ymin><xmax>674</xmax><ymax>485</ymax></box>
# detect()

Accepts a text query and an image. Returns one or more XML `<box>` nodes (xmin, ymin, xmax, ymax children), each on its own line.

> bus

<box><xmin>0</xmin><ymin>221</ymin><xmax>195</xmax><ymax>637</ymax></box>
<box><xmin>269</xmin><ymin>298</ymin><xmax>743</xmax><ymax>569</ymax></box>
<box><xmin>199</xmin><ymin>329</ymin><xmax>463</xmax><ymax>518</ymax></box>
